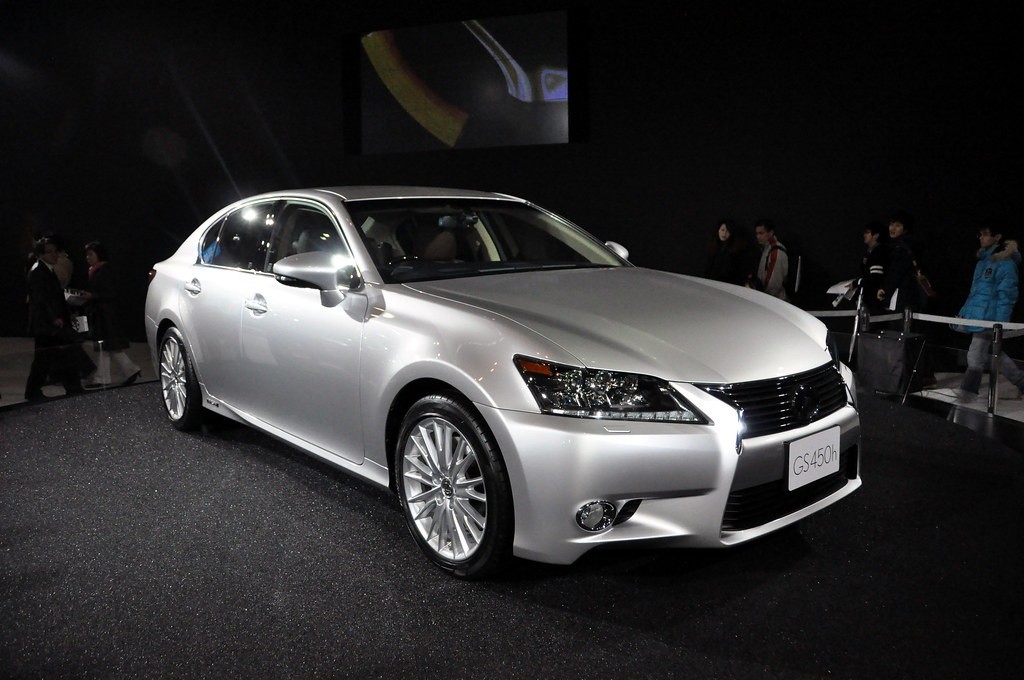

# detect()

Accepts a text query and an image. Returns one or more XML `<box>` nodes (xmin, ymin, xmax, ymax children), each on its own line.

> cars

<box><xmin>145</xmin><ymin>187</ymin><xmax>862</xmax><ymax>580</ymax></box>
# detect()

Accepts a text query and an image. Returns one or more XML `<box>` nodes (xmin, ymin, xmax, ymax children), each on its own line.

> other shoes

<box><xmin>903</xmin><ymin>378</ymin><xmax>925</xmax><ymax>393</ymax></box>
<box><xmin>66</xmin><ymin>388</ymin><xmax>89</xmax><ymax>398</ymax></box>
<box><xmin>916</xmin><ymin>376</ymin><xmax>937</xmax><ymax>389</ymax></box>
<box><xmin>26</xmin><ymin>390</ymin><xmax>50</xmax><ymax>402</ymax></box>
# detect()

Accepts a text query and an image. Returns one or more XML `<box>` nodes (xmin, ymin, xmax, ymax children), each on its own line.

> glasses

<box><xmin>976</xmin><ymin>232</ymin><xmax>992</xmax><ymax>239</ymax></box>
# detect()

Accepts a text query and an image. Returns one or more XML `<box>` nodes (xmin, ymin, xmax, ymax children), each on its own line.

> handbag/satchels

<box><xmin>917</xmin><ymin>273</ymin><xmax>936</xmax><ymax>296</ymax></box>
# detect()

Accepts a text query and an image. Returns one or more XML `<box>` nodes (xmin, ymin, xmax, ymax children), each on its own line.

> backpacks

<box><xmin>764</xmin><ymin>246</ymin><xmax>795</xmax><ymax>288</ymax></box>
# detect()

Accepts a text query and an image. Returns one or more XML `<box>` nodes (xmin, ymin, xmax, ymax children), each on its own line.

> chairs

<box><xmin>296</xmin><ymin>222</ymin><xmax>379</xmax><ymax>267</ymax></box>
<box><xmin>412</xmin><ymin>225</ymin><xmax>457</xmax><ymax>267</ymax></box>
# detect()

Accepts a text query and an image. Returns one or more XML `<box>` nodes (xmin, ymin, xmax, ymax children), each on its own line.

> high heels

<box><xmin>86</xmin><ymin>383</ymin><xmax>112</xmax><ymax>388</ymax></box>
<box><xmin>123</xmin><ymin>371</ymin><xmax>143</xmax><ymax>384</ymax></box>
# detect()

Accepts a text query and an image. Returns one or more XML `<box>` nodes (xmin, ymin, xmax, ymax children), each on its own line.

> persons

<box><xmin>951</xmin><ymin>223</ymin><xmax>1024</xmax><ymax>400</ymax></box>
<box><xmin>707</xmin><ymin>218</ymin><xmax>742</xmax><ymax>283</ymax></box>
<box><xmin>742</xmin><ymin>221</ymin><xmax>788</xmax><ymax>300</ymax></box>
<box><xmin>24</xmin><ymin>237</ymin><xmax>85</xmax><ymax>401</ymax></box>
<box><xmin>85</xmin><ymin>241</ymin><xmax>142</xmax><ymax>388</ymax></box>
<box><xmin>843</xmin><ymin>208</ymin><xmax>915</xmax><ymax>331</ymax></box>
